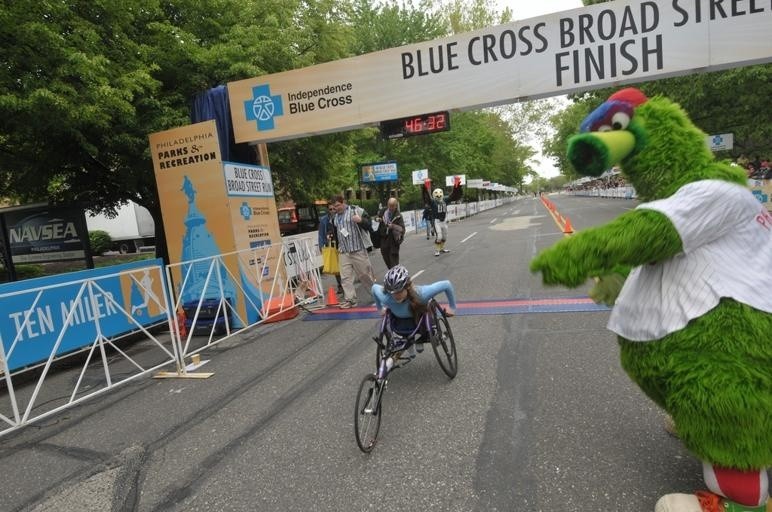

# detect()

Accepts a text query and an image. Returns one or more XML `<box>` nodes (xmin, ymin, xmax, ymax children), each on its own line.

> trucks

<box><xmin>84</xmin><ymin>194</ymin><xmax>154</xmax><ymax>255</ymax></box>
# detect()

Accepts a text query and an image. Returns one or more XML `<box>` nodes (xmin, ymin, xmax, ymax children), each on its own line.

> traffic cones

<box><xmin>327</xmin><ymin>286</ymin><xmax>340</xmax><ymax>306</ymax></box>
<box><xmin>539</xmin><ymin>191</ymin><xmax>574</xmax><ymax>235</ymax></box>
<box><xmin>425</xmin><ymin>178</ymin><xmax>432</xmax><ymax>190</ymax></box>
<box><xmin>454</xmin><ymin>176</ymin><xmax>461</xmax><ymax>188</ymax></box>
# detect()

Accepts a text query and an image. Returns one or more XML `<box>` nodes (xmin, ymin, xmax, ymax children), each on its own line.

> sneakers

<box><xmin>340</xmin><ymin>301</ymin><xmax>358</xmax><ymax>309</ymax></box>
<box><xmin>337</xmin><ymin>286</ymin><xmax>342</xmax><ymax>295</ymax></box>
<box><xmin>434</xmin><ymin>247</ymin><xmax>450</xmax><ymax>256</ymax></box>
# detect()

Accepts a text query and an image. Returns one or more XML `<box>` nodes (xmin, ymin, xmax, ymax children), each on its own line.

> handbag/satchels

<box><xmin>321</xmin><ymin>237</ymin><xmax>340</xmax><ymax>276</ymax></box>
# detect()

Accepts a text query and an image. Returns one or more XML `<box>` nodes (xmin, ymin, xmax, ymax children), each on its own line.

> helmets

<box><xmin>384</xmin><ymin>263</ymin><xmax>409</xmax><ymax>294</ymax></box>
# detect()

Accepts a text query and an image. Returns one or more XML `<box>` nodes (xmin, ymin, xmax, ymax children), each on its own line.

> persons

<box><xmin>318</xmin><ymin>199</ymin><xmax>344</xmax><ymax>294</ymax></box>
<box><xmin>565</xmin><ymin>153</ymin><xmax>771</xmax><ymax>192</ymax></box>
<box><xmin>421</xmin><ymin>177</ymin><xmax>464</xmax><ymax>256</ymax></box>
<box><xmin>374</xmin><ymin>197</ymin><xmax>406</xmax><ymax>268</ymax></box>
<box><xmin>422</xmin><ymin>204</ymin><xmax>434</xmax><ymax>241</ymax></box>
<box><xmin>371</xmin><ymin>264</ymin><xmax>457</xmax><ymax>357</ymax></box>
<box><xmin>325</xmin><ymin>195</ymin><xmax>377</xmax><ymax>309</ymax></box>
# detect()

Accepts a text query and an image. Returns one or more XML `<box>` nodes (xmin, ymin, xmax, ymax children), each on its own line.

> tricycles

<box><xmin>353</xmin><ymin>292</ymin><xmax>460</xmax><ymax>455</ymax></box>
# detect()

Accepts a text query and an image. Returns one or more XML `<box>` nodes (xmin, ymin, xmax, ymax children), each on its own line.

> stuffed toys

<box><xmin>527</xmin><ymin>86</ymin><xmax>772</xmax><ymax>512</ymax></box>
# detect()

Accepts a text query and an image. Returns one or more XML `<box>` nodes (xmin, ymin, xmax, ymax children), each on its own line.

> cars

<box><xmin>278</xmin><ymin>198</ymin><xmax>332</xmax><ymax>237</ymax></box>
